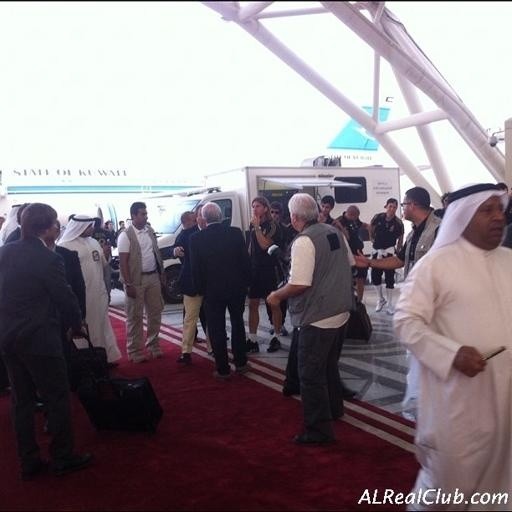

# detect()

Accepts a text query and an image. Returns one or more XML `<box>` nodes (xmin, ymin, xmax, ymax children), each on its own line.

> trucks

<box><xmin>99</xmin><ymin>156</ymin><xmax>403</xmax><ymax>303</ymax></box>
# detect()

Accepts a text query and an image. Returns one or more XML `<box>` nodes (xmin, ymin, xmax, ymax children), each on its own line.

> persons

<box><xmin>0</xmin><ymin>183</ymin><xmax>512</xmax><ymax>414</ymax></box>
<box><xmin>0</xmin><ymin>202</ymin><xmax>98</xmax><ymax>479</ymax></box>
<box><xmin>393</xmin><ymin>184</ymin><xmax>511</xmax><ymax>510</ymax></box>
<box><xmin>267</xmin><ymin>193</ymin><xmax>358</xmax><ymax>445</ymax></box>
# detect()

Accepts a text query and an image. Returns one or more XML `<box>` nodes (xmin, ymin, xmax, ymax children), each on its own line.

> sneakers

<box><xmin>214</xmin><ymin>371</ymin><xmax>244</xmax><ymax>381</ymax></box>
<box><xmin>152</xmin><ymin>350</ymin><xmax>163</xmax><ymax>360</ymax></box>
<box><xmin>267</xmin><ymin>336</ymin><xmax>281</xmax><ymax>352</ymax></box>
<box><xmin>176</xmin><ymin>353</ymin><xmax>191</xmax><ymax>362</ymax></box>
<box><xmin>128</xmin><ymin>351</ymin><xmax>147</xmax><ymax>363</ymax></box>
<box><xmin>387</xmin><ymin>305</ymin><xmax>394</xmax><ymax>315</ymax></box>
<box><xmin>376</xmin><ymin>299</ymin><xmax>387</xmax><ymax>312</ymax></box>
<box><xmin>245</xmin><ymin>339</ymin><xmax>260</xmax><ymax>353</ymax></box>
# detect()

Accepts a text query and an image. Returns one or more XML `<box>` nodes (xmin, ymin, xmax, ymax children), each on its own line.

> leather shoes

<box><xmin>293</xmin><ymin>433</ymin><xmax>335</xmax><ymax>442</ymax></box>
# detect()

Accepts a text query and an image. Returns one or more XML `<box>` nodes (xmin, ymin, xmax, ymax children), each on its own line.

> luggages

<box><xmin>342</xmin><ymin>300</ymin><xmax>373</xmax><ymax>341</ymax></box>
<box><xmin>73</xmin><ymin>320</ymin><xmax>134</xmax><ymax>429</ymax></box>
<box><xmin>68</xmin><ymin>322</ymin><xmax>106</xmax><ymax>391</ymax></box>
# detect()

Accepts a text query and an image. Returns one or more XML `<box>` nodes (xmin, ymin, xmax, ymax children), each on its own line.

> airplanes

<box><xmin>1</xmin><ymin>89</ymin><xmax>394</xmax><ymax>245</ymax></box>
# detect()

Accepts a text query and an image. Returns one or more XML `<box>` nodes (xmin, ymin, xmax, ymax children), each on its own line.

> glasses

<box><xmin>401</xmin><ymin>203</ymin><xmax>411</xmax><ymax>206</ymax></box>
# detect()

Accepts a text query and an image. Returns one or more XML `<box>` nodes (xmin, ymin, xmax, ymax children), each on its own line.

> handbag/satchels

<box><xmin>114</xmin><ymin>377</ymin><xmax>162</xmax><ymax>431</ymax></box>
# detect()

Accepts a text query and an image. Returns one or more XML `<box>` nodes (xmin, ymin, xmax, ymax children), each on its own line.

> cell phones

<box><xmin>480</xmin><ymin>345</ymin><xmax>505</xmax><ymax>360</ymax></box>
